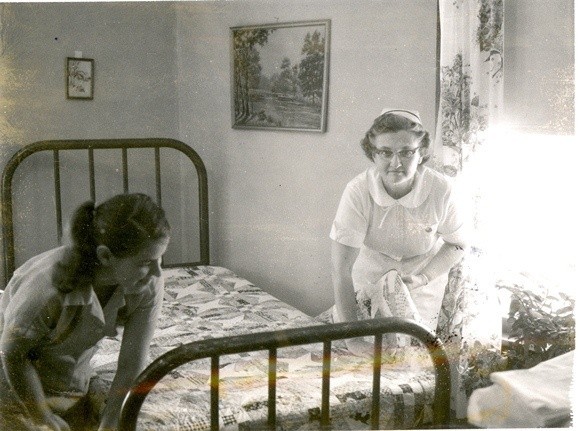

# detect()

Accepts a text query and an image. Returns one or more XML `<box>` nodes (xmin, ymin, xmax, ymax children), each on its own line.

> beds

<box><xmin>0</xmin><ymin>137</ymin><xmax>451</xmax><ymax>430</ymax></box>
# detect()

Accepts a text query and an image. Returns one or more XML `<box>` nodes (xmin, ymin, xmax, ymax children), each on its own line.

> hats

<box><xmin>381</xmin><ymin>108</ymin><xmax>421</xmax><ymax>125</ymax></box>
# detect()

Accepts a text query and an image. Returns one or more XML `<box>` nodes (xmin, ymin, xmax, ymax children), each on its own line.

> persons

<box><xmin>330</xmin><ymin>107</ymin><xmax>468</xmax><ymax>366</ymax></box>
<box><xmin>0</xmin><ymin>193</ymin><xmax>172</xmax><ymax>431</ymax></box>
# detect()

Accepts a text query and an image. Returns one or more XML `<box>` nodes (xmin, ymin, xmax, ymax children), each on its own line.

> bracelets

<box><xmin>421</xmin><ymin>272</ymin><xmax>430</xmax><ymax>286</ymax></box>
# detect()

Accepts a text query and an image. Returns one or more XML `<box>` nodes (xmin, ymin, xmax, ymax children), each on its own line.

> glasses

<box><xmin>368</xmin><ymin>145</ymin><xmax>426</xmax><ymax>159</ymax></box>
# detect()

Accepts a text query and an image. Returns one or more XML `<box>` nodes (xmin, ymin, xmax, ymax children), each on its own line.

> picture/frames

<box><xmin>65</xmin><ymin>57</ymin><xmax>94</xmax><ymax>100</ymax></box>
<box><xmin>228</xmin><ymin>19</ymin><xmax>332</xmax><ymax>133</ymax></box>
